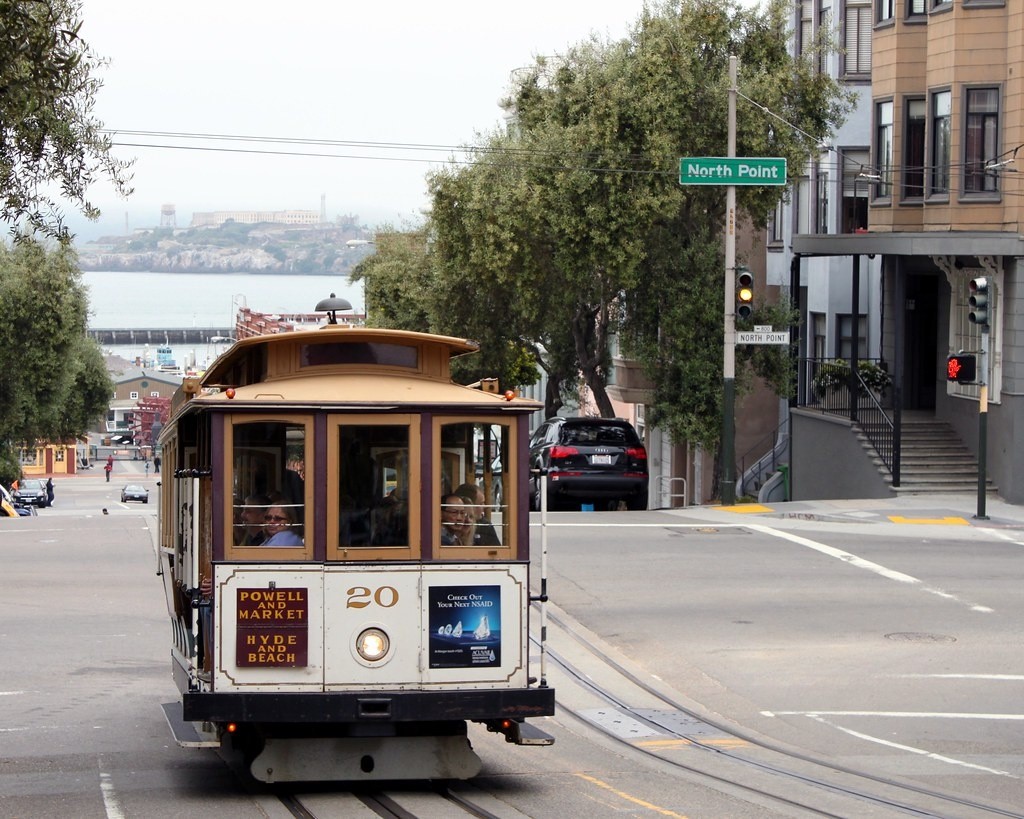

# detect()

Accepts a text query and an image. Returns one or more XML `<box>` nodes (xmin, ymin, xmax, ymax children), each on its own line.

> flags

<box><xmin>11</xmin><ymin>480</ymin><xmax>17</xmax><ymax>490</ymax></box>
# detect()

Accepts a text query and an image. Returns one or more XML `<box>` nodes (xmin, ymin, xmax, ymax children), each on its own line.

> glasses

<box><xmin>264</xmin><ymin>514</ymin><xmax>287</xmax><ymax>522</ymax></box>
<box><xmin>443</xmin><ymin>509</ymin><xmax>468</xmax><ymax>518</ymax></box>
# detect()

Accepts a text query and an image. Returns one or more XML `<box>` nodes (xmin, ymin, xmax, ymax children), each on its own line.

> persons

<box><xmin>153</xmin><ymin>454</ymin><xmax>161</xmax><ymax>473</ymax></box>
<box><xmin>339</xmin><ymin>496</ymin><xmax>408</xmax><ymax>546</ymax></box>
<box><xmin>107</xmin><ymin>455</ymin><xmax>113</xmax><ymax>467</ymax></box>
<box><xmin>46</xmin><ymin>477</ymin><xmax>54</xmax><ymax>507</ymax></box>
<box><xmin>144</xmin><ymin>460</ymin><xmax>150</xmax><ymax>477</ymax></box>
<box><xmin>234</xmin><ymin>431</ymin><xmax>305</xmax><ymax>546</ymax></box>
<box><xmin>440</xmin><ymin>483</ymin><xmax>501</xmax><ymax>547</ymax></box>
<box><xmin>104</xmin><ymin>463</ymin><xmax>112</xmax><ymax>482</ymax></box>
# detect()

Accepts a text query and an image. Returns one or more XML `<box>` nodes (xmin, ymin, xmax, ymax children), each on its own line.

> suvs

<box><xmin>529</xmin><ymin>415</ymin><xmax>651</xmax><ymax>513</ymax></box>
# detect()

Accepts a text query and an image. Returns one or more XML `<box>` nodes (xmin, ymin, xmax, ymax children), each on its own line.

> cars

<box><xmin>120</xmin><ymin>484</ymin><xmax>149</xmax><ymax>503</ymax></box>
<box><xmin>13</xmin><ymin>479</ymin><xmax>47</xmax><ymax>509</ymax></box>
<box><xmin>478</xmin><ymin>452</ymin><xmax>502</xmax><ymax>512</ymax></box>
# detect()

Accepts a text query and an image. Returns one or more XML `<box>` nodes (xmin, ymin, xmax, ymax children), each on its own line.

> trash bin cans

<box><xmin>777</xmin><ymin>462</ymin><xmax>789</xmax><ymax>501</ymax></box>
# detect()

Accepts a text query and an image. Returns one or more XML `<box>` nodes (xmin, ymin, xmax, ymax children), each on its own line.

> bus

<box><xmin>156</xmin><ymin>294</ymin><xmax>556</xmax><ymax>785</ymax></box>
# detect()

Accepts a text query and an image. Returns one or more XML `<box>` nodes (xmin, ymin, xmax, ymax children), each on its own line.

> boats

<box><xmin>131</xmin><ymin>341</ymin><xmax>183</xmax><ymax>377</ymax></box>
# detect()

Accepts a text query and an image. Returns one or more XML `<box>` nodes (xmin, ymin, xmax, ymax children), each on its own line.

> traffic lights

<box><xmin>737</xmin><ymin>270</ymin><xmax>752</xmax><ymax>318</ymax></box>
<box><xmin>968</xmin><ymin>275</ymin><xmax>992</xmax><ymax>327</ymax></box>
<box><xmin>947</xmin><ymin>355</ymin><xmax>976</xmax><ymax>381</ymax></box>
